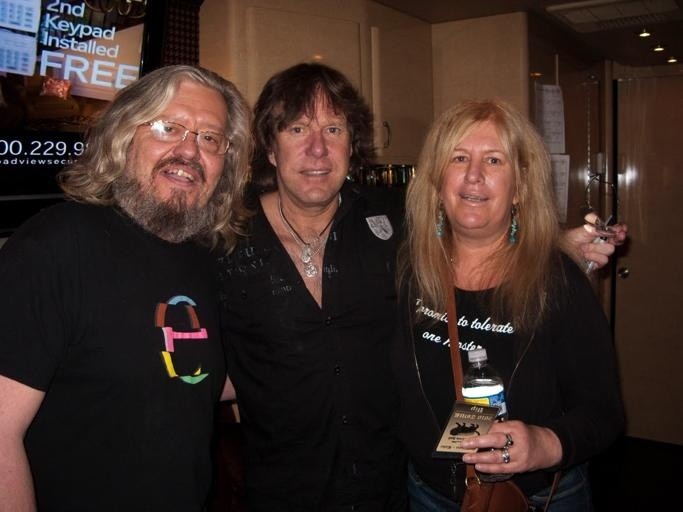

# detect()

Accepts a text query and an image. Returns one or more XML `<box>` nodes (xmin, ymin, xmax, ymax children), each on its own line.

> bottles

<box><xmin>347</xmin><ymin>163</ymin><xmax>416</xmax><ymax>186</ymax></box>
<box><xmin>461</xmin><ymin>348</ymin><xmax>514</xmax><ymax>483</ymax></box>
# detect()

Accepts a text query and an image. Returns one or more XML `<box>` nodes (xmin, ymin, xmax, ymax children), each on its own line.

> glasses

<box><xmin>138</xmin><ymin>121</ymin><xmax>235</xmax><ymax>155</ymax></box>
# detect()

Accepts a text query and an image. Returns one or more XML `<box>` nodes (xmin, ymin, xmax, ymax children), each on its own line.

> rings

<box><xmin>503</xmin><ymin>433</ymin><xmax>513</xmax><ymax>448</ymax></box>
<box><xmin>501</xmin><ymin>449</ymin><xmax>510</xmax><ymax>463</ymax></box>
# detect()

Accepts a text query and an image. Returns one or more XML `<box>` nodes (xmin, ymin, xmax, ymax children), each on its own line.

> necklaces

<box><xmin>278</xmin><ymin>192</ymin><xmax>342</xmax><ymax>279</ymax></box>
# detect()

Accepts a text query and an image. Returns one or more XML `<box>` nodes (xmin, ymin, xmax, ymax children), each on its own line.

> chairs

<box><xmin>7</xmin><ymin>61</ymin><xmax>79</xmax><ymax>126</ymax></box>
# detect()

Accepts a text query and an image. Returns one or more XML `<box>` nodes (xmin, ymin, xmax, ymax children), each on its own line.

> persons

<box><xmin>388</xmin><ymin>98</ymin><xmax>625</xmax><ymax>512</ymax></box>
<box><xmin>207</xmin><ymin>61</ymin><xmax>629</xmax><ymax>512</ymax></box>
<box><xmin>0</xmin><ymin>65</ymin><xmax>257</xmax><ymax>512</ymax></box>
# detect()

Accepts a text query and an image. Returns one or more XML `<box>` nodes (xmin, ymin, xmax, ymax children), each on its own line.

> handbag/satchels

<box><xmin>462</xmin><ymin>477</ymin><xmax>530</xmax><ymax>512</ymax></box>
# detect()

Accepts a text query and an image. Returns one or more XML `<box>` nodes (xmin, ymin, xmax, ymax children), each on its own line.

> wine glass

<box><xmin>581</xmin><ymin>190</ymin><xmax>629</xmax><ymax>281</ymax></box>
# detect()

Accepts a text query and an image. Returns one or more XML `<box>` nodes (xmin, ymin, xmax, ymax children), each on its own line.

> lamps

<box><xmin>637</xmin><ymin>8</ymin><xmax>677</xmax><ymax>63</ymax></box>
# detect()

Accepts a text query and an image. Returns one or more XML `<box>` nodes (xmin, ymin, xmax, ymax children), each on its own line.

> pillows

<box><xmin>39</xmin><ymin>76</ymin><xmax>72</xmax><ymax>100</ymax></box>
